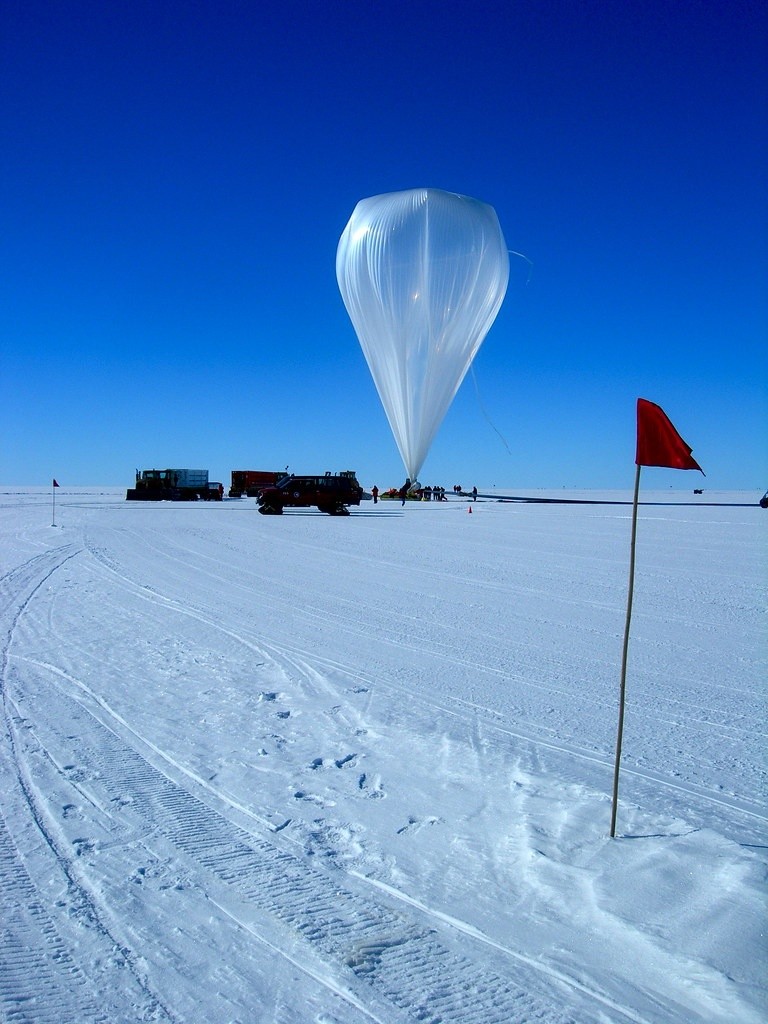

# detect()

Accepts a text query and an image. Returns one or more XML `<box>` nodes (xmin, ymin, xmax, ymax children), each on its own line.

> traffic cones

<box><xmin>468</xmin><ymin>506</ymin><xmax>473</xmax><ymax>513</ymax></box>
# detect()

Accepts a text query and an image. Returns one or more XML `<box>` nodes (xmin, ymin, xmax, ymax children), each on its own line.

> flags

<box><xmin>635</xmin><ymin>398</ymin><xmax>705</xmax><ymax>475</ymax></box>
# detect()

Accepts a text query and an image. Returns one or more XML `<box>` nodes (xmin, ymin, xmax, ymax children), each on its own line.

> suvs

<box><xmin>256</xmin><ymin>473</ymin><xmax>364</xmax><ymax>506</ymax></box>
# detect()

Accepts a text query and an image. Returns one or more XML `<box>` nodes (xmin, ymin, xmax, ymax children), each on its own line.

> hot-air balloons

<box><xmin>337</xmin><ymin>190</ymin><xmax>511</xmax><ymax>502</ymax></box>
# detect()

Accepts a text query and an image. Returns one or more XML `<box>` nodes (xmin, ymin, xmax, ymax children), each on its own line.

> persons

<box><xmin>400</xmin><ymin>488</ymin><xmax>409</xmax><ymax>506</ymax></box>
<box><xmin>472</xmin><ymin>487</ymin><xmax>477</xmax><ymax>501</ymax></box>
<box><xmin>218</xmin><ymin>484</ymin><xmax>224</xmax><ymax>501</ymax></box>
<box><xmin>371</xmin><ymin>486</ymin><xmax>378</xmax><ymax>503</ymax></box>
<box><xmin>453</xmin><ymin>485</ymin><xmax>462</xmax><ymax>492</ymax></box>
<box><xmin>424</xmin><ymin>486</ymin><xmax>447</xmax><ymax>500</ymax></box>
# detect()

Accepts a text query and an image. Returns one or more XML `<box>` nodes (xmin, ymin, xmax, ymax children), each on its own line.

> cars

<box><xmin>203</xmin><ymin>481</ymin><xmax>224</xmax><ymax>500</ymax></box>
